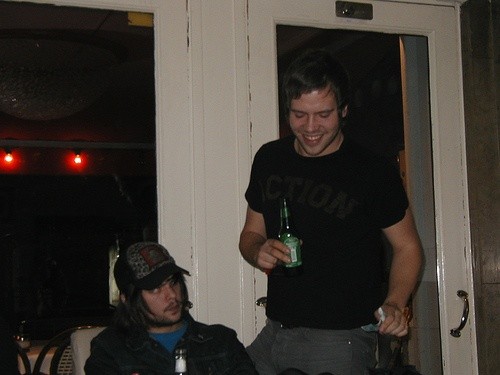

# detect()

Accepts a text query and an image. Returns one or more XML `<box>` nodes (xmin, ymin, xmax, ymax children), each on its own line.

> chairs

<box><xmin>32</xmin><ymin>326</ymin><xmax>106</xmax><ymax>375</ymax></box>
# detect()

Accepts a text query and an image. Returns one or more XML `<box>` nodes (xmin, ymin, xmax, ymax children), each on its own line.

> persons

<box><xmin>238</xmin><ymin>48</ymin><xmax>424</xmax><ymax>375</ymax></box>
<box><xmin>84</xmin><ymin>241</ymin><xmax>260</xmax><ymax>375</ymax></box>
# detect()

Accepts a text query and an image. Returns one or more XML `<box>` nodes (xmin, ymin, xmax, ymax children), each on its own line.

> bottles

<box><xmin>174</xmin><ymin>349</ymin><xmax>188</xmax><ymax>375</ymax></box>
<box><xmin>278</xmin><ymin>195</ymin><xmax>303</xmax><ymax>277</ymax></box>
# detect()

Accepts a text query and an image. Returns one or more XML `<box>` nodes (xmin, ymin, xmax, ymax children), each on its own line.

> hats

<box><xmin>113</xmin><ymin>242</ymin><xmax>191</xmax><ymax>290</ymax></box>
